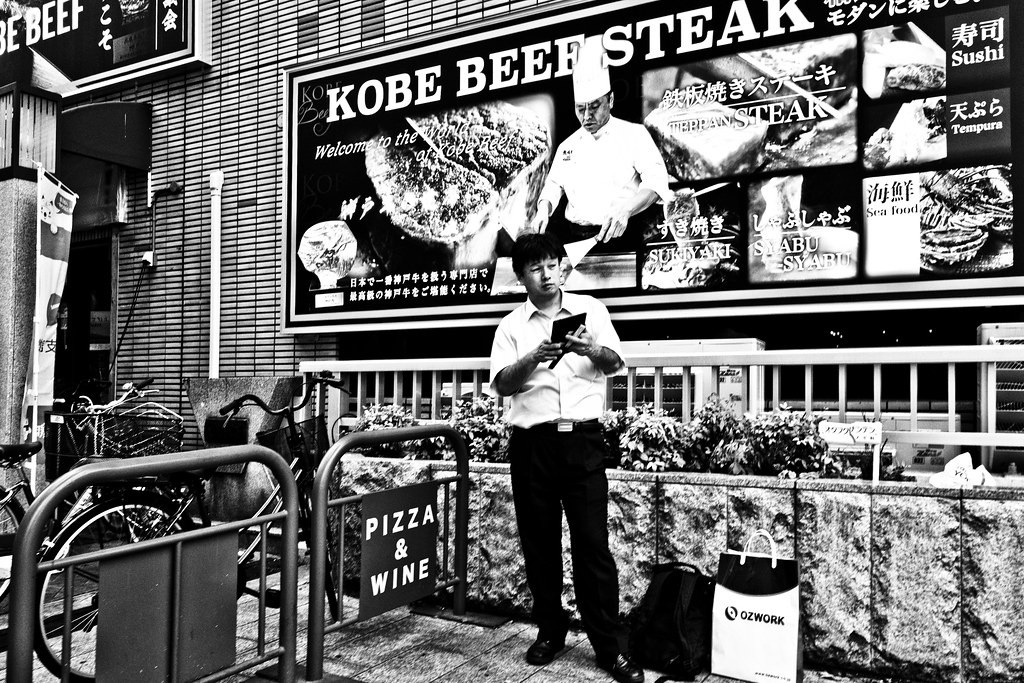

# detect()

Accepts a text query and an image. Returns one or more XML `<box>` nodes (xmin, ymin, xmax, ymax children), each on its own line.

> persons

<box><xmin>531</xmin><ymin>71</ymin><xmax>672</xmax><ymax>251</ymax></box>
<box><xmin>485</xmin><ymin>233</ymin><xmax>645</xmax><ymax>683</ymax></box>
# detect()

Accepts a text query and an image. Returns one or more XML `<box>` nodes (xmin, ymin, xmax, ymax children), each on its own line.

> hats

<box><xmin>573</xmin><ymin>34</ymin><xmax>611</xmax><ymax>103</ymax></box>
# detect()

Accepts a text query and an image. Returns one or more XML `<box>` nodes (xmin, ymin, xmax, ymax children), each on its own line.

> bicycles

<box><xmin>2</xmin><ymin>375</ymin><xmax>341</xmax><ymax>683</ymax></box>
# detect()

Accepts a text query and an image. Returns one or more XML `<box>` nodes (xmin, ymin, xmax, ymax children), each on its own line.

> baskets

<box><xmin>45</xmin><ymin>410</ymin><xmax>185</xmax><ymax>482</ymax></box>
<box><xmin>256</xmin><ymin>412</ymin><xmax>329</xmax><ymax>473</ymax></box>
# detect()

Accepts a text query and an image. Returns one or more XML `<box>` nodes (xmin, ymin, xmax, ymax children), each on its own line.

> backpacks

<box><xmin>629</xmin><ymin>562</ymin><xmax>715</xmax><ymax>683</ymax></box>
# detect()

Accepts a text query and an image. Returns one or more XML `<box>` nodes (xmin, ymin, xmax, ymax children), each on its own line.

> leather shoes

<box><xmin>526</xmin><ymin>632</ymin><xmax>565</xmax><ymax>665</ymax></box>
<box><xmin>596</xmin><ymin>651</ymin><xmax>645</xmax><ymax>683</ymax></box>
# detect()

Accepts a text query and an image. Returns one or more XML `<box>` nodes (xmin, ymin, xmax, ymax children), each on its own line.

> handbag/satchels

<box><xmin>711</xmin><ymin>528</ymin><xmax>803</xmax><ymax>683</ymax></box>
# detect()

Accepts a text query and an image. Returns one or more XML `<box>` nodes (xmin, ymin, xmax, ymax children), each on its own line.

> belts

<box><xmin>513</xmin><ymin>418</ymin><xmax>604</xmax><ymax>434</ymax></box>
